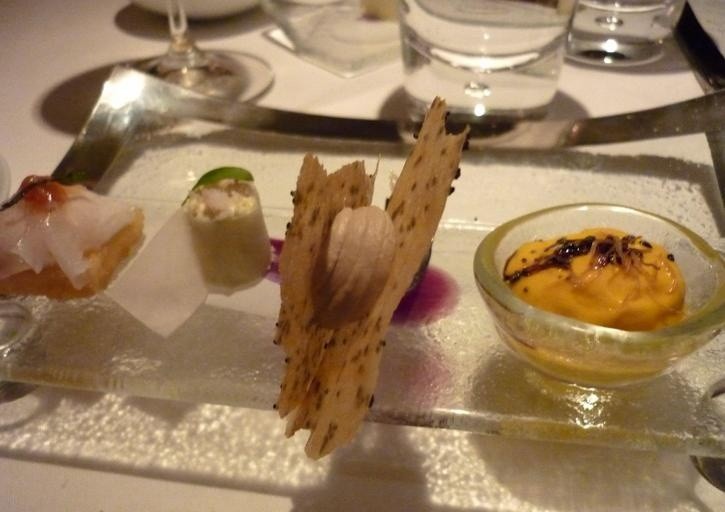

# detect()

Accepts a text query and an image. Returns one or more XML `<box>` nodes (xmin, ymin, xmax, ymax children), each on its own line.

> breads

<box><xmin>273</xmin><ymin>98</ymin><xmax>472</xmax><ymax>459</ymax></box>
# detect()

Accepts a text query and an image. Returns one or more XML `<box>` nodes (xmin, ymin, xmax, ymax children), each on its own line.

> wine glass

<box><xmin>116</xmin><ymin>0</ymin><xmax>282</xmax><ymax>116</ymax></box>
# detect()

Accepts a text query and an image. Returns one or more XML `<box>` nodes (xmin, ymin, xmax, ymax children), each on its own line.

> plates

<box><xmin>0</xmin><ymin>101</ymin><xmax>725</xmax><ymax>458</ymax></box>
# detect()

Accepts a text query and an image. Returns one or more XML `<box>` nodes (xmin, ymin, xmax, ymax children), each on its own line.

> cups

<box><xmin>554</xmin><ymin>0</ymin><xmax>690</xmax><ymax>70</ymax></box>
<box><xmin>471</xmin><ymin>199</ymin><xmax>724</xmax><ymax>392</ymax></box>
<box><xmin>397</xmin><ymin>0</ymin><xmax>568</xmax><ymax>124</ymax></box>
<box><xmin>264</xmin><ymin>2</ymin><xmax>406</xmax><ymax>76</ymax></box>
